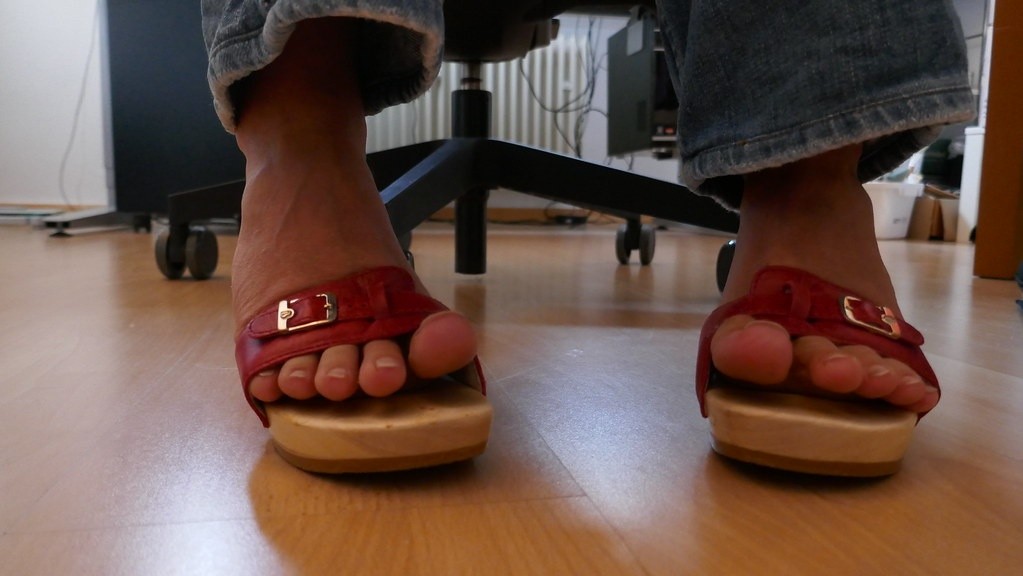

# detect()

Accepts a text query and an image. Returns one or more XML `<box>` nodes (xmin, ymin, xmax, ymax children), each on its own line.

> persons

<box><xmin>198</xmin><ymin>0</ymin><xmax>980</xmax><ymax>476</ymax></box>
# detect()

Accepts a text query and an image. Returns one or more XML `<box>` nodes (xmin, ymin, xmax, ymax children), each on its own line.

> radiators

<box><xmin>365</xmin><ymin>39</ymin><xmax>600</xmax><ymax>230</ymax></box>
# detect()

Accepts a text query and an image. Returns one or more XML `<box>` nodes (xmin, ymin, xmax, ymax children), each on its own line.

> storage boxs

<box><xmin>907</xmin><ymin>182</ymin><xmax>961</xmax><ymax>241</ymax></box>
<box><xmin>861</xmin><ymin>180</ymin><xmax>925</xmax><ymax>240</ymax></box>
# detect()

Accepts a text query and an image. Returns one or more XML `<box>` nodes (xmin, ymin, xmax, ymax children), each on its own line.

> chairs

<box><xmin>148</xmin><ymin>0</ymin><xmax>746</xmax><ymax>298</ymax></box>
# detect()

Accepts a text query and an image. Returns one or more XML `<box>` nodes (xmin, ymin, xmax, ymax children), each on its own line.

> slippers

<box><xmin>235</xmin><ymin>266</ymin><xmax>492</xmax><ymax>473</ymax></box>
<box><xmin>689</xmin><ymin>265</ymin><xmax>948</xmax><ymax>478</ymax></box>
